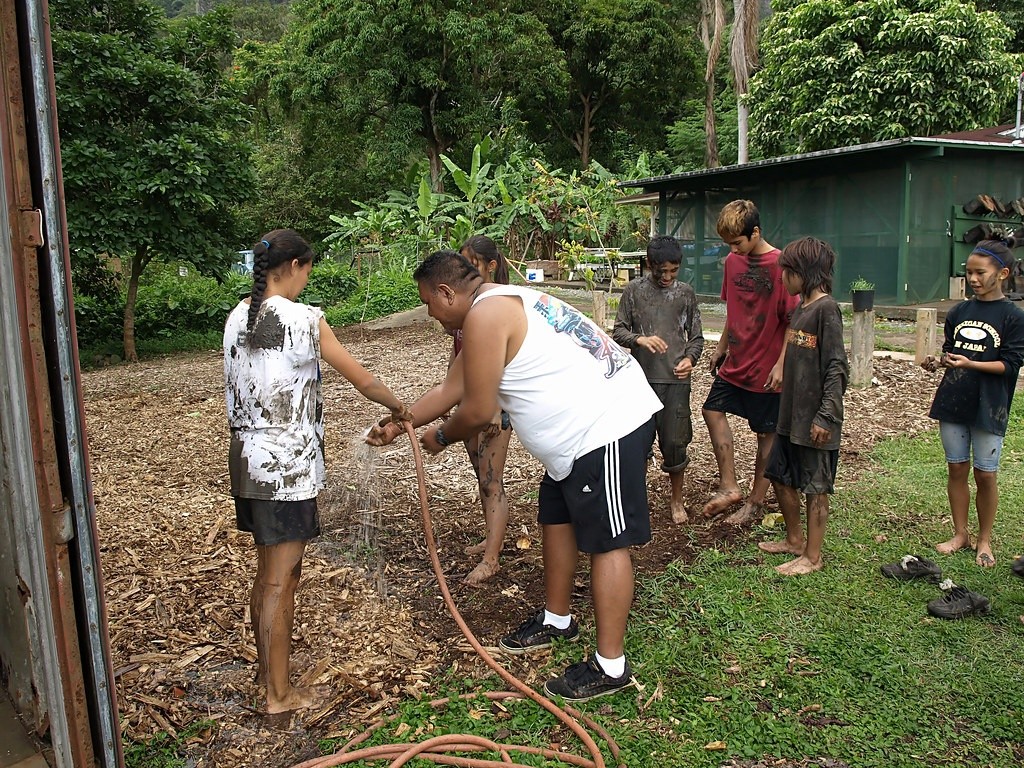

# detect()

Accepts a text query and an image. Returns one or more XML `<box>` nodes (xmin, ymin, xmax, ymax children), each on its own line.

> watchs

<box><xmin>435</xmin><ymin>425</ymin><xmax>448</xmax><ymax>447</ymax></box>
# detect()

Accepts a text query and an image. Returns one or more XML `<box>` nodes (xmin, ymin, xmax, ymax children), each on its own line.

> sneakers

<box><xmin>499</xmin><ymin>607</ymin><xmax>579</xmax><ymax>654</ymax></box>
<box><xmin>544</xmin><ymin>657</ymin><xmax>635</xmax><ymax>704</ymax></box>
<box><xmin>928</xmin><ymin>582</ymin><xmax>986</xmax><ymax>617</ymax></box>
<box><xmin>880</xmin><ymin>554</ymin><xmax>941</xmax><ymax>578</ymax></box>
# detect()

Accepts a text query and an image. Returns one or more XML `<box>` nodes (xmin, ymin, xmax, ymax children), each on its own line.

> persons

<box><xmin>701</xmin><ymin>200</ymin><xmax>801</xmax><ymax>525</ymax></box>
<box><xmin>441</xmin><ymin>235</ymin><xmax>510</xmax><ymax>584</ymax></box>
<box><xmin>612</xmin><ymin>235</ymin><xmax>705</xmax><ymax>525</ymax></box>
<box><xmin>920</xmin><ymin>240</ymin><xmax>1024</xmax><ymax>568</ymax></box>
<box><xmin>366</xmin><ymin>250</ymin><xmax>666</xmax><ymax>701</ymax></box>
<box><xmin>757</xmin><ymin>237</ymin><xmax>850</xmax><ymax>576</ymax></box>
<box><xmin>221</xmin><ymin>229</ymin><xmax>413</xmax><ymax>714</ymax></box>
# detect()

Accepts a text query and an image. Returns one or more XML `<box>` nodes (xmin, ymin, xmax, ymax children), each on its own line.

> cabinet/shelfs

<box><xmin>951</xmin><ymin>204</ymin><xmax>1024</xmax><ymax>300</ymax></box>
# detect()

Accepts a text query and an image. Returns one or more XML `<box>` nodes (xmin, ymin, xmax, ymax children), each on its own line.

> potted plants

<box><xmin>848</xmin><ymin>276</ymin><xmax>875</xmax><ymax>311</ymax></box>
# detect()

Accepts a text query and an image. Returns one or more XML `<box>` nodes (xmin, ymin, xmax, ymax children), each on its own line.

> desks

<box><xmin>595</xmin><ymin>252</ymin><xmax>647</xmax><ymax>284</ymax></box>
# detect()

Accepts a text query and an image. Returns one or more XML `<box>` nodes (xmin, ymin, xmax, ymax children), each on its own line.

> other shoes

<box><xmin>1012</xmin><ymin>556</ymin><xmax>1024</xmax><ymax>577</ymax></box>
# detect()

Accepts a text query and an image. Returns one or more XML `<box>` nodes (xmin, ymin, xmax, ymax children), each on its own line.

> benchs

<box><xmin>561</xmin><ymin>248</ymin><xmax>619</xmax><ymax>281</ymax></box>
